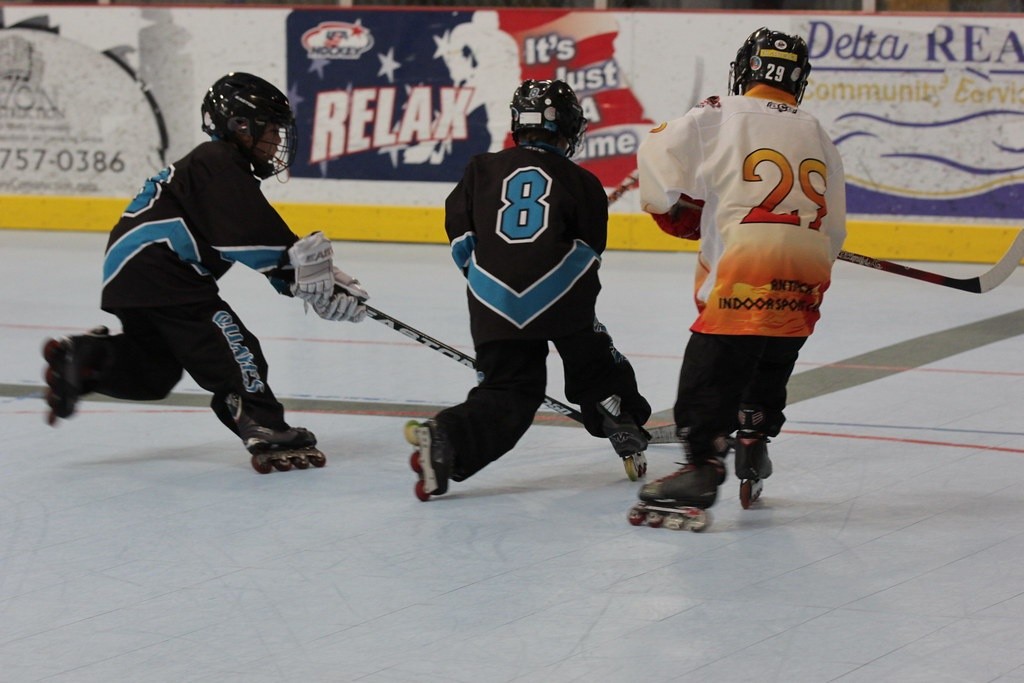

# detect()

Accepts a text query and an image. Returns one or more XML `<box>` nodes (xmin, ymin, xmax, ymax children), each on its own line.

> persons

<box><xmin>628</xmin><ymin>26</ymin><xmax>848</xmax><ymax>534</ymax></box>
<box><xmin>41</xmin><ymin>70</ymin><xmax>371</xmax><ymax>475</ymax></box>
<box><xmin>405</xmin><ymin>76</ymin><xmax>654</xmax><ymax>502</ymax></box>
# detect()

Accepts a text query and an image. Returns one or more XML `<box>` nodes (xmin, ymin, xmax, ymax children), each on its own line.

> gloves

<box><xmin>653</xmin><ymin>192</ymin><xmax>705</xmax><ymax>241</ymax></box>
<box><xmin>288</xmin><ymin>230</ymin><xmax>336</xmax><ymax>308</ymax></box>
<box><xmin>313</xmin><ymin>265</ymin><xmax>370</xmax><ymax>323</ymax></box>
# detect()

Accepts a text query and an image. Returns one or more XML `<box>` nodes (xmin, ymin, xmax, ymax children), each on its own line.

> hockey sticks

<box><xmin>836</xmin><ymin>227</ymin><xmax>1024</xmax><ymax>295</ymax></box>
<box><xmin>333</xmin><ymin>285</ymin><xmax>687</xmax><ymax>444</ymax></box>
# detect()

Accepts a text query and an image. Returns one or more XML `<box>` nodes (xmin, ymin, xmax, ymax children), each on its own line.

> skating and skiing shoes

<box><xmin>225</xmin><ymin>393</ymin><xmax>327</xmax><ymax>474</ymax></box>
<box><xmin>609</xmin><ymin>423</ymin><xmax>649</xmax><ymax>482</ymax></box>
<box><xmin>44</xmin><ymin>325</ymin><xmax>110</xmax><ymax>424</ymax></box>
<box><xmin>734</xmin><ymin>405</ymin><xmax>773</xmax><ymax>509</ymax></box>
<box><xmin>628</xmin><ymin>435</ymin><xmax>729</xmax><ymax>532</ymax></box>
<box><xmin>403</xmin><ymin>416</ymin><xmax>448</xmax><ymax>501</ymax></box>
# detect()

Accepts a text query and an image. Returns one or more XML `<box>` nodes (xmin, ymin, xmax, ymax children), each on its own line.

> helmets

<box><xmin>727</xmin><ymin>27</ymin><xmax>811</xmax><ymax>104</ymax></box>
<box><xmin>202</xmin><ymin>72</ymin><xmax>297</xmax><ymax>178</ymax></box>
<box><xmin>509</xmin><ymin>79</ymin><xmax>586</xmax><ymax>158</ymax></box>
